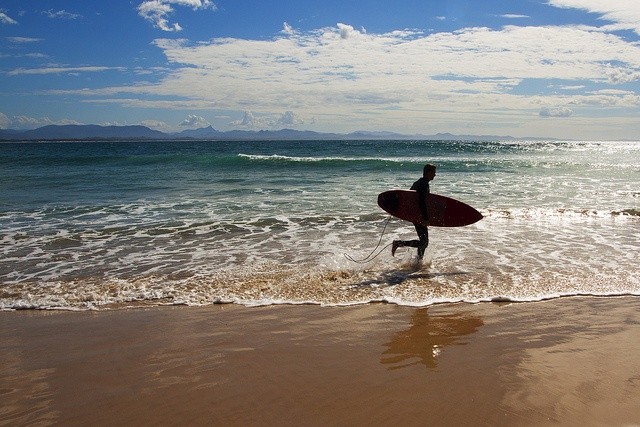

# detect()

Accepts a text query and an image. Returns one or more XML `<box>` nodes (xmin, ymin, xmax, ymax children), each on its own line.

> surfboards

<box><xmin>378</xmin><ymin>190</ymin><xmax>484</xmax><ymax>227</ymax></box>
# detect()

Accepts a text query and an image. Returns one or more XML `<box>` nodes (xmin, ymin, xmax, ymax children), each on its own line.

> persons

<box><xmin>392</xmin><ymin>163</ymin><xmax>437</xmax><ymax>261</ymax></box>
